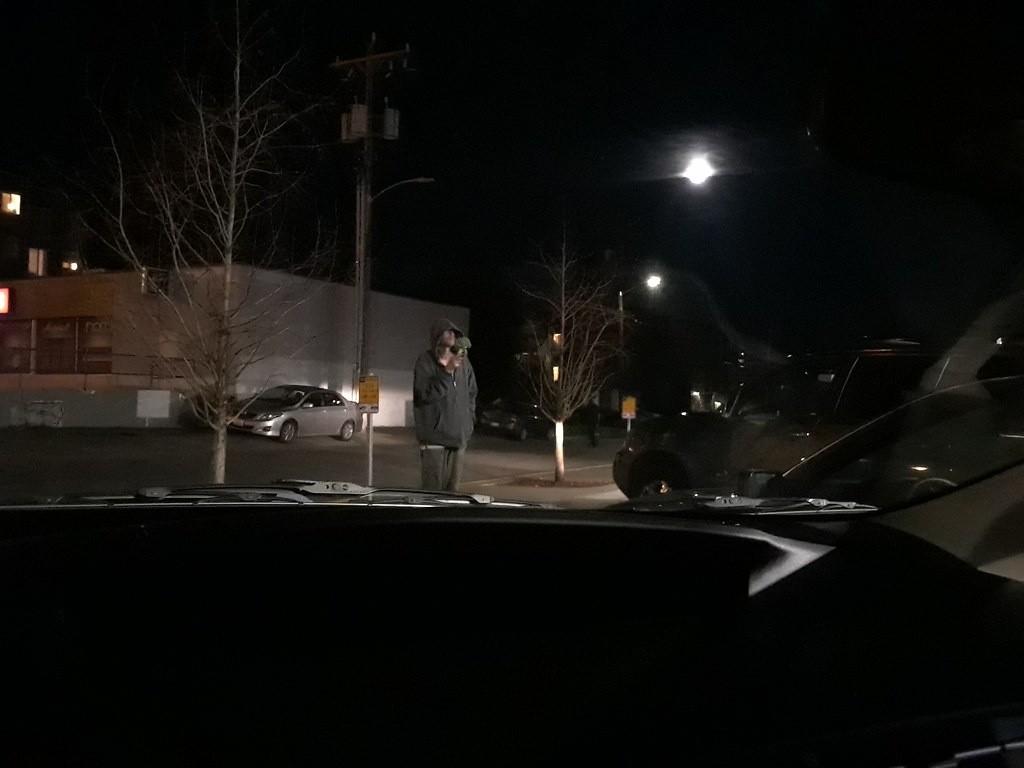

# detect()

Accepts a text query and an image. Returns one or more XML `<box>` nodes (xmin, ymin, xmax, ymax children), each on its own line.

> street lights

<box><xmin>332</xmin><ymin>36</ymin><xmax>437</xmax><ymax>425</ymax></box>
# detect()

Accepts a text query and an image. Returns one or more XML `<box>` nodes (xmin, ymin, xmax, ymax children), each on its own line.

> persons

<box><xmin>584</xmin><ymin>399</ymin><xmax>598</xmax><ymax>447</ymax></box>
<box><xmin>413</xmin><ymin>318</ymin><xmax>477</xmax><ymax>493</ymax></box>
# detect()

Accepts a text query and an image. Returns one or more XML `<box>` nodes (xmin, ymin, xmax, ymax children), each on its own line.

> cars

<box><xmin>480</xmin><ymin>398</ymin><xmax>555</xmax><ymax>442</ymax></box>
<box><xmin>226</xmin><ymin>384</ymin><xmax>364</xmax><ymax>444</ymax></box>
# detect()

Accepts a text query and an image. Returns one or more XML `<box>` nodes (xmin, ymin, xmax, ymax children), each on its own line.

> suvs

<box><xmin>610</xmin><ymin>336</ymin><xmax>1021</xmax><ymax>513</ymax></box>
